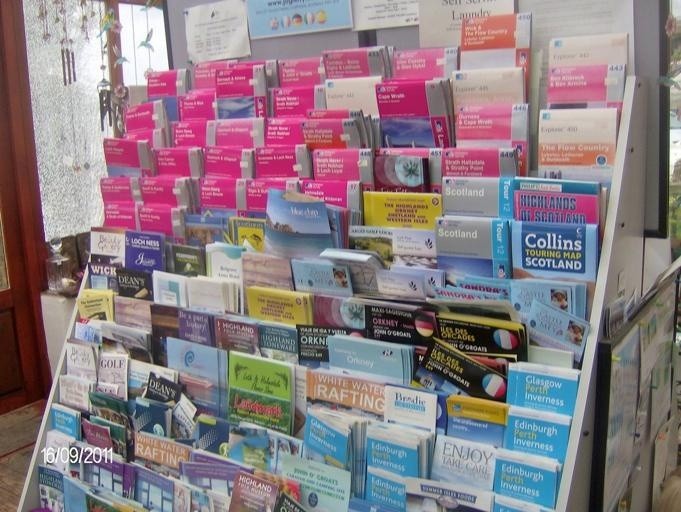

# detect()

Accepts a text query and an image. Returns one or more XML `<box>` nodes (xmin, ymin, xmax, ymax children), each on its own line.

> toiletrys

<box><xmin>45</xmin><ymin>237</ymin><xmax>71</xmax><ymax>294</ymax></box>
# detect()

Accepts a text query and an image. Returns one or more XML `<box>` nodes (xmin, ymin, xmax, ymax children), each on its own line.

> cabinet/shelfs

<box><xmin>2</xmin><ymin>52</ymin><xmax>648</xmax><ymax>512</ymax></box>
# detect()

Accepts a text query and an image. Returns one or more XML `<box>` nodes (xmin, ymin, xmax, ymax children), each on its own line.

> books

<box><xmin>100</xmin><ymin>69</ymin><xmax>189</xmax><ymax>236</ymax></box>
<box><xmin>142</xmin><ymin>60</ymin><xmax>237</xmax><ymax>242</ymax></box>
<box><xmin>230</xmin><ymin>56</ymin><xmax>324</xmax><ymax>247</ymax></box>
<box><xmin>188</xmin><ymin>58</ymin><xmax>277</xmax><ymax>244</ymax></box>
<box><xmin>279</xmin><ymin>44</ymin><xmax>394</xmax><ymax>257</ymax></box>
<box><xmin>510</xmin><ymin>32</ymin><xmax>628</xmax><ymax>334</ymax></box>
<box><xmin>340</xmin><ymin>45</ymin><xmax>458</xmax><ymax>266</ymax></box>
<box><xmin>436</xmin><ymin>11</ymin><xmax>533</xmax><ymax>277</ymax></box>
